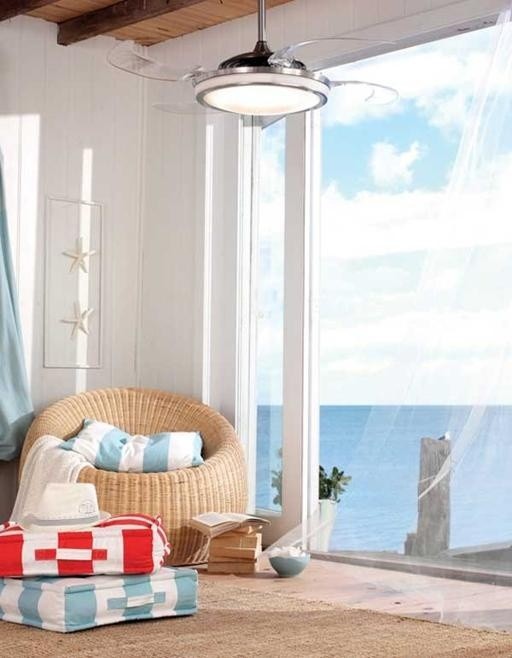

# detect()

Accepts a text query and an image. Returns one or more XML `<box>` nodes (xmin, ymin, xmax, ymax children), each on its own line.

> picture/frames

<box><xmin>42</xmin><ymin>195</ymin><xmax>105</xmax><ymax>370</ymax></box>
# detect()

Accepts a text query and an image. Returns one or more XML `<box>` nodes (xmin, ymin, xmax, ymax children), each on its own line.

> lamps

<box><xmin>194</xmin><ymin>72</ymin><xmax>329</xmax><ymax>117</ymax></box>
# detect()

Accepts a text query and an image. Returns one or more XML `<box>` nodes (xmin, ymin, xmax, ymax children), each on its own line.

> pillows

<box><xmin>59</xmin><ymin>417</ymin><xmax>206</xmax><ymax>471</ymax></box>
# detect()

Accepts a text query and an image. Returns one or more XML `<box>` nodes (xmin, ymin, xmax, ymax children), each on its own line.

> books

<box><xmin>189</xmin><ymin>510</ymin><xmax>271</xmax><ymax>573</ymax></box>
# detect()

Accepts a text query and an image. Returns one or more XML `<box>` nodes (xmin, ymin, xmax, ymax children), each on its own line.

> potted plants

<box><xmin>268</xmin><ymin>446</ymin><xmax>353</xmax><ymax>553</ymax></box>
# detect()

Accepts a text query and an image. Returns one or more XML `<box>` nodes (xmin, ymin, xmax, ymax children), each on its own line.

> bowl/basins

<box><xmin>269</xmin><ymin>554</ymin><xmax>312</xmax><ymax>578</ymax></box>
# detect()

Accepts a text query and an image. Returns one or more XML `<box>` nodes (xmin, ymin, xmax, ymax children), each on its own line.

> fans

<box><xmin>106</xmin><ymin>0</ymin><xmax>401</xmax><ymax>129</ymax></box>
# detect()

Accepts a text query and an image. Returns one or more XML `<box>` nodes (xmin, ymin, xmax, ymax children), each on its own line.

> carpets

<box><xmin>0</xmin><ymin>567</ymin><xmax>512</xmax><ymax>658</ymax></box>
